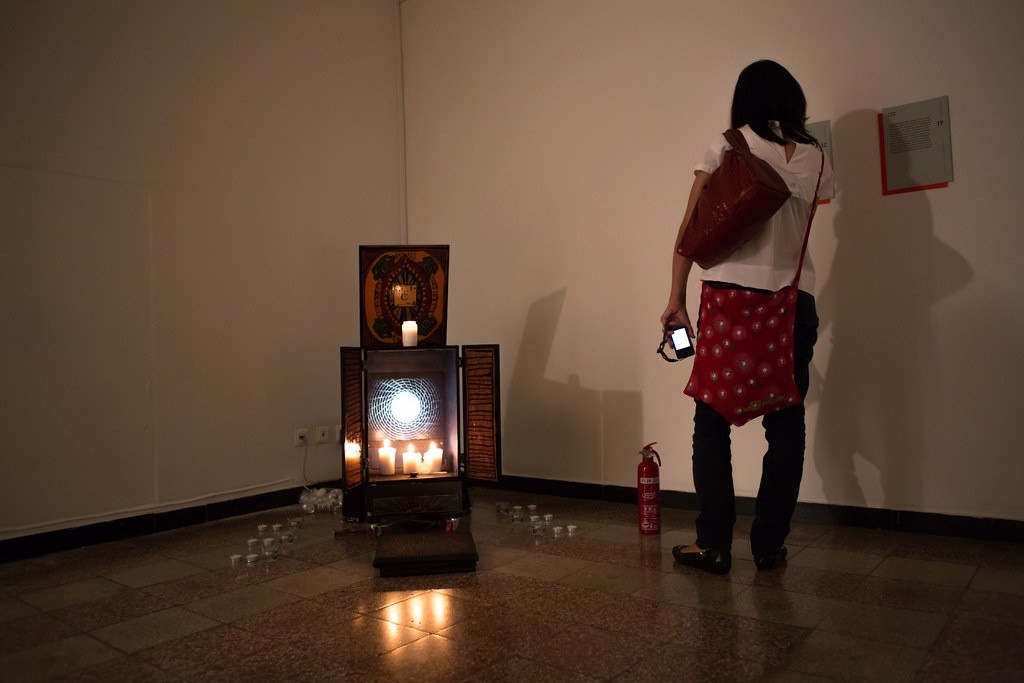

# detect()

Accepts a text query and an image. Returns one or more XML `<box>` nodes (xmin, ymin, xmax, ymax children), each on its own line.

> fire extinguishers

<box><xmin>638</xmin><ymin>442</ymin><xmax>663</xmax><ymax>536</ymax></box>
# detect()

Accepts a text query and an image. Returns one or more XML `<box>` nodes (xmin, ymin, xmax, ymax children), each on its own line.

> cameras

<box><xmin>670</xmin><ymin>326</ymin><xmax>695</xmax><ymax>359</ymax></box>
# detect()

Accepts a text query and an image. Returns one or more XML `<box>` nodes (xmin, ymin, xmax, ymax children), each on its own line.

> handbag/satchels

<box><xmin>677</xmin><ymin>127</ymin><xmax>792</xmax><ymax>269</ymax></box>
<box><xmin>683</xmin><ymin>283</ymin><xmax>801</xmax><ymax>427</ymax></box>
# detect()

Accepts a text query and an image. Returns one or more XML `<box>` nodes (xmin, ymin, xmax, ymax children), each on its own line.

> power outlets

<box><xmin>294</xmin><ymin>428</ymin><xmax>308</xmax><ymax>447</ymax></box>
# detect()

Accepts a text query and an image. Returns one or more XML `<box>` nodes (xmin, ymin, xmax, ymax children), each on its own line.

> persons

<box><xmin>660</xmin><ymin>60</ymin><xmax>835</xmax><ymax>573</ymax></box>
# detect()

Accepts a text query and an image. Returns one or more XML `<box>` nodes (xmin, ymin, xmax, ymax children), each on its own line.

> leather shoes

<box><xmin>673</xmin><ymin>544</ymin><xmax>731</xmax><ymax>575</ymax></box>
<box><xmin>754</xmin><ymin>545</ymin><xmax>787</xmax><ymax>570</ymax></box>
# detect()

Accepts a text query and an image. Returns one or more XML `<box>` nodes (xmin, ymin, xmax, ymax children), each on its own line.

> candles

<box><xmin>401</xmin><ymin>320</ymin><xmax>418</xmax><ymax>346</ymax></box>
<box><xmin>378</xmin><ymin>438</ymin><xmax>396</xmax><ymax>476</ymax></box>
<box><xmin>401</xmin><ymin>443</ymin><xmax>420</xmax><ymax>473</ymax></box>
<box><xmin>423</xmin><ymin>441</ymin><xmax>444</xmax><ymax>471</ymax></box>
